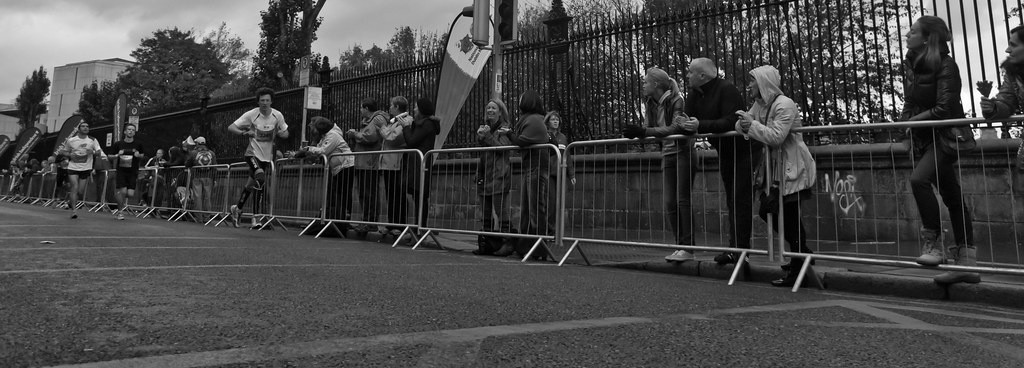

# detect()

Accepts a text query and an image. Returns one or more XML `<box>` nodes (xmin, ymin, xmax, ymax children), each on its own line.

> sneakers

<box><xmin>379</xmin><ymin>227</ymin><xmax>390</xmax><ymax>235</ymax></box>
<box><xmin>665</xmin><ymin>249</ymin><xmax>695</xmax><ymax>261</ymax></box>
<box><xmin>713</xmin><ymin>251</ymin><xmax>750</xmax><ymax>264</ymax></box>
<box><xmin>251</xmin><ymin>217</ymin><xmax>262</xmax><ymax>229</ymax></box>
<box><xmin>230</xmin><ymin>204</ymin><xmax>243</xmax><ymax>228</ymax></box>
<box><xmin>390</xmin><ymin>229</ymin><xmax>401</xmax><ymax>236</ymax></box>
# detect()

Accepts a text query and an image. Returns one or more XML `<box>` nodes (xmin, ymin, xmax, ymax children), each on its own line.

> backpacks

<box><xmin>194</xmin><ymin>148</ymin><xmax>214</xmax><ymax>172</ymax></box>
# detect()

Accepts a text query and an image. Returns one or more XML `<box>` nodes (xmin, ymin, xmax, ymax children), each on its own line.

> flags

<box><xmin>55</xmin><ymin>115</ymin><xmax>85</xmax><ymax>161</ymax></box>
<box><xmin>431</xmin><ymin>6</ymin><xmax>500</xmax><ymax>164</ymax></box>
<box><xmin>113</xmin><ymin>92</ymin><xmax>127</xmax><ymax>141</ymax></box>
<box><xmin>0</xmin><ymin>135</ymin><xmax>10</xmax><ymax>157</ymax></box>
<box><xmin>11</xmin><ymin>127</ymin><xmax>42</xmax><ymax>164</ymax></box>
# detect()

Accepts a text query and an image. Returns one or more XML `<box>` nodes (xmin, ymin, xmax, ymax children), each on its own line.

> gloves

<box><xmin>621</xmin><ymin>124</ymin><xmax>645</xmax><ymax>139</ymax></box>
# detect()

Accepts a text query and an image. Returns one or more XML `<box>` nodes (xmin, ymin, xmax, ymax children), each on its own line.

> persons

<box><xmin>346</xmin><ymin>96</ymin><xmax>413</xmax><ymax>235</ymax></box>
<box><xmin>62</xmin><ymin>122</ymin><xmax>102</xmax><ymax>218</ymax></box>
<box><xmin>286</xmin><ymin>116</ymin><xmax>355</xmax><ymax>234</ymax></box>
<box><xmin>621</xmin><ymin>68</ymin><xmax>696</xmax><ymax>261</ymax></box>
<box><xmin>397</xmin><ymin>98</ymin><xmax>441</xmax><ymax>245</ymax></box>
<box><xmin>979</xmin><ymin>26</ymin><xmax>1024</xmax><ymax>124</ymax></box>
<box><xmin>228</xmin><ymin>88</ymin><xmax>289</xmax><ymax>229</ymax></box>
<box><xmin>144</xmin><ymin>137</ymin><xmax>217</xmax><ymax>224</ymax></box>
<box><xmin>470</xmin><ymin>97</ymin><xmax>515</xmax><ymax>257</ymax></box>
<box><xmin>675</xmin><ymin>58</ymin><xmax>753</xmax><ymax>270</ymax></box>
<box><xmin>108</xmin><ymin>123</ymin><xmax>146</xmax><ymax>220</ymax></box>
<box><xmin>500</xmin><ymin>89</ymin><xmax>569</xmax><ymax>257</ymax></box>
<box><xmin>2</xmin><ymin>156</ymin><xmax>58</xmax><ymax>205</ymax></box>
<box><xmin>735</xmin><ymin>66</ymin><xmax>817</xmax><ymax>284</ymax></box>
<box><xmin>902</xmin><ymin>16</ymin><xmax>982</xmax><ymax>286</ymax></box>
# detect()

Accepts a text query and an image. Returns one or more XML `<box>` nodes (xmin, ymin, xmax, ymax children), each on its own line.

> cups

<box><xmin>349</xmin><ymin>129</ymin><xmax>355</xmax><ymax>132</ymax></box>
<box><xmin>301</xmin><ymin>141</ymin><xmax>309</xmax><ymax>147</ymax></box>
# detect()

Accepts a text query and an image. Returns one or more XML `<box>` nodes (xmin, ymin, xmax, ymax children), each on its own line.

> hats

<box><xmin>193</xmin><ymin>136</ymin><xmax>206</xmax><ymax>144</ymax></box>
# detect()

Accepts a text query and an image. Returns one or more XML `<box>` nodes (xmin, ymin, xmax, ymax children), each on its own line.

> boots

<box><xmin>472</xmin><ymin>219</ymin><xmax>492</xmax><ymax>256</ymax></box>
<box><xmin>933</xmin><ymin>245</ymin><xmax>981</xmax><ymax>286</ymax></box>
<box><xmin>492</xmin><ymin>220</ymin><xmax>514</xmax><ymax>257</ymax></box>
<box><xmin>916</xmin><ymin>225</ymin><xmax>949</xmax><ymax>265</ymax></box>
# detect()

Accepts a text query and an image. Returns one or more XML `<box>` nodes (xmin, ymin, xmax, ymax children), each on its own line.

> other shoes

<box><xmin>354</xmin><ymin>224</ymin><xmax>378</xmax><ymax>234</ymax></box>
<box><xmin>770</xmin><ymin>269</ymin><xmax>809</xmax><ymax>287</ymax></box>
<box><xmin>71</xmin><ymin>211</ymin><xmax>78</xmax><ymax>219</ymax></box>
<box><xmin>115</xmin><ymin>212</ymin><xmax>125</xmax><ymax>220</ymax></box>
<box><xmin>779</xmin><ymin>249</ymin><xmax>816</xmax><ymax>270</ymax></box>
